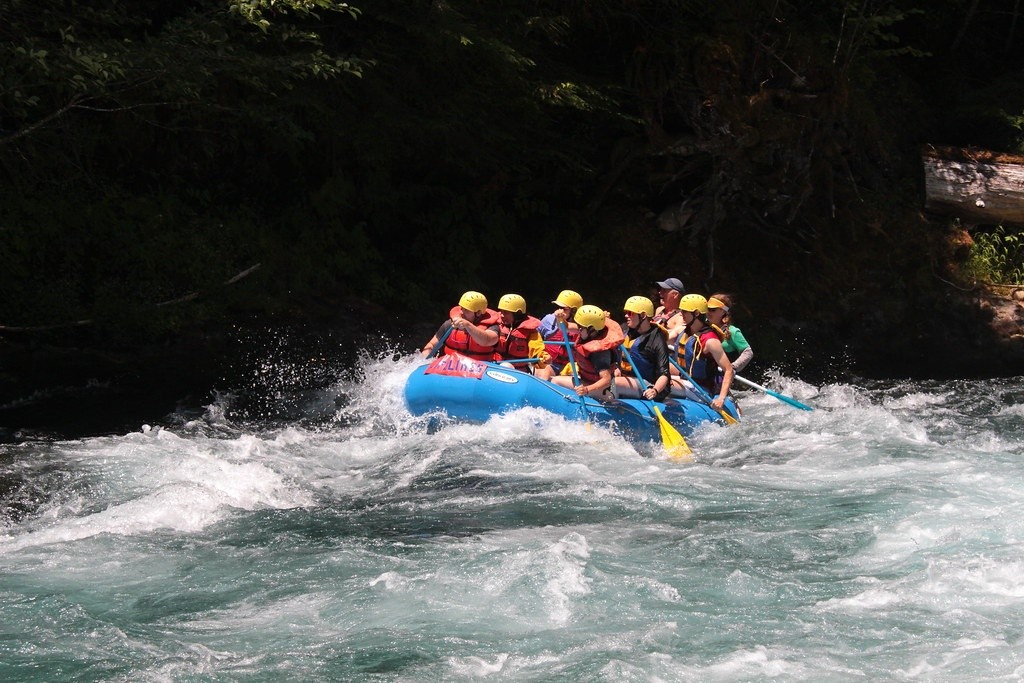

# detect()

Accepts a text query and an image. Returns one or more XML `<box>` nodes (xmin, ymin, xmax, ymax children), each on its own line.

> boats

<box><xmin>400</xmin><ymin>355</ymin><xmax>741</xmax><ymax>441</ymax></box>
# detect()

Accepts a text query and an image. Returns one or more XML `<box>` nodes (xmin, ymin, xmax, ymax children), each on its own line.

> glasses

<box><xmin>679</xmin><ymin>310</ymin><xmax>693</xmax><ymax>316</ymax></box>
<box><xmin>624</xmin><ymin>311</ymin><xmax>641</xmax><ymax>316</ymax></box>
<box><xmin>558</xmin><ymin>306</ymin><xmax>570</xmax><ymax>311</ymax></box>
<box><xmin>577</xmin><ymin>324</ymin><xmax>587</xmax><ymax>331</ymax></box>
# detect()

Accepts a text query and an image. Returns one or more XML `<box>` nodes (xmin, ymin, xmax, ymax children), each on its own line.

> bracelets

<box><xmin>586</xmin><ymin>387</ymin><xmax>588</xmax><ymax>395</ymax></box>
<box><xmin>653</xmin><ymin>388</ymin><xmax>659</xmax><ymax>396</ymax></box>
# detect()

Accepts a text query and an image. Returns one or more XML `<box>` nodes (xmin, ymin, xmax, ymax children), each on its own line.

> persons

<box><xmin>669</xmin><ymin>294</ymin><xmax>734</xmax><ymax>411</ymax></box>
<box><xmin>533</xmin><ymin>290</ymin><xmax>584</xmax><ymax>381</ymax></box>
<box><xmin>493</xmin><ymin>294</ymin><xmax>552</xmax><ymax>375</ymax></box>
<box><xmin>609</xmin><ymin>296</ymin><xmax>671</xmax><ymax>402</ymax></box>
<box><xmin>705</xmin><ymin>294</ymin><xmax>754</xmax><ymax>376</ymax></box>
<box><xmin>547</xmin><ymin>305</ymin><xmax>617</xmax><ymax>404</ymax></box>
<box><xmin>421</xmin><ymin>291</ymin><xmax>500</xmax><ymax>362</ymax></box>
<box><xmin>650</xmin><ymin>278</ymin><xmax>686</xmax><ymax>362</ymax></box>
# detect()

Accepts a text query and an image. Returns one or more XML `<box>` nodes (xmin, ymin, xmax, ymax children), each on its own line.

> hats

<box><xmin>655</xmin><ymin>278</ymin><xmax>685</xmax><ymax>294</ymax></box>
<box><xmin>707</xmin><ymin>297</ymin><xmax>729</xmax><ymax>312</ymax></box>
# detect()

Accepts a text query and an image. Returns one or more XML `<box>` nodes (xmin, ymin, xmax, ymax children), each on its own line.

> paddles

<box><xmin>668</xmin><ymin>356</ymin><xmax>741</xmax><ymax>429</ymax></box>
<box><xmin>487</xmin><ymin>357</ymin><xmax>544</xmax><ymax>365</ymax></box>
<box><xmin>427</xmin><ymin>324</ymin><xmax>455</xmax><ymax>359</ymax></box>
<box><xmin>558</xmin><ymin>313</ymin><xmax>592</xmax><ymax>424</ymax></box>
<box><xmin>620</xmin><ymin>344</ymin><xmax>692</xmax><ymax>456</ymax></box>
<box><xmin>718</xmin><ymin>366</ymin><xmax>812</xmax><ymax>411</ymax></box>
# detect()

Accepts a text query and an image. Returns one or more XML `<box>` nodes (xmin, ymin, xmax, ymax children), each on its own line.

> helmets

<box><xmin>679</xmin><ymin>294</ymin><xmax>709</xmax><ymax>314</ymax></box>
<box><xmin>573</xmin><ymin>305</ymin><xmax>606</xmax><ymax>330</ymax></box>
<box><xmin>498</xmin><ymin>293</ymin><xmax>527</xmax><ymax>314</ymax></box>
<box><xmin>623</xmin><ymin>295</ymin><xmax>654</xmax><ymax>317</ymax></box>
<box><xmin>551</xmin><ymin>290</ymin><xmax>583</xmax><ymax>310</ymax></box>
<box><xmin>458</xmin><ymin>291</ymin><xmax>488</xmax><ymax>314</ymax></box>
